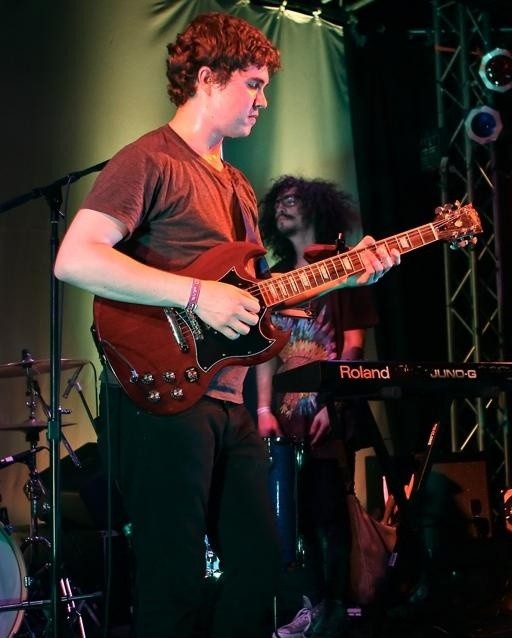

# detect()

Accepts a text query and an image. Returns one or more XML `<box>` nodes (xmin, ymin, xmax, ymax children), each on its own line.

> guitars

<box><xmin>93</xmin><ymin>199</ymin><xmax>483</xmax><ymax>415</ymax></box>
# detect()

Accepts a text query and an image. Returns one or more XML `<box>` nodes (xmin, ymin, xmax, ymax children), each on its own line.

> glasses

<box><xmin>273</xmin><ymin>195</ymin><xmax>302</xmax><ymax>207</ymax></box>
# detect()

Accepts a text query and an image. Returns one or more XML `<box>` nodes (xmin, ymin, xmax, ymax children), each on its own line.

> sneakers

<box><xmin>273</xmin><ymin>597</ymin><xmax>345</xmax><ymax>638</ymax></box>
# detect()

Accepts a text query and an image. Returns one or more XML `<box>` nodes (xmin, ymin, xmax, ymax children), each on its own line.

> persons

<box><xmin>469</xmin><ymin>498</ymin><xmax>490</xmax><ymax>539</ymax></box>
<box><xmin>245</xmin><ymin>172</ymin><xmax>382</xmax><ymax>638</ymax></box>
<box><xmin>51</xmin><ymin>10</ymin><xmax>403</xmax><ymax>638</ymax></box>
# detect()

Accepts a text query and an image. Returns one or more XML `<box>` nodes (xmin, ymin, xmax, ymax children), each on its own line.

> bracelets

<box><xmin>255</xmin><ymin>406</ymin><xmax>272</xmax><ymax>416</ymax></box>
<box><xmin>183</xmin><ymin>277</ymin><xmax>202</xmax><ymax>313</ymax></box>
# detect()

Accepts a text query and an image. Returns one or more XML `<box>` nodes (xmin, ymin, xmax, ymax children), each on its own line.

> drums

<box><xmin>1</xmin><ymin>521</ymin><xmax>64</xmax><ymax>638</ymax></box>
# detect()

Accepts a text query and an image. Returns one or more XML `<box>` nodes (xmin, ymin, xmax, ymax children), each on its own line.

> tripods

<box><xmin>18</xmin><ymin>442</ymin><xmax>102</xmax><ymax>638</ymax></box>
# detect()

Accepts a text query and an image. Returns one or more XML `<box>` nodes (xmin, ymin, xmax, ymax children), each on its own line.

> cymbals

<box><xmin>0</xmin><ymin>359</ymin><xmax>89</xmax><ymax>378</ymax></box>
<box><xmin>0</xmin><ymin>421</ymin><xmax>76</xmax><ymax>431</ymax></box>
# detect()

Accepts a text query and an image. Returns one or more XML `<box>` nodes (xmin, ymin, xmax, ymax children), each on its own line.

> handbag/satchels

<box><xmin>347</xmin><ymin>494</ymin><xmax>399</xmax><ymax>606</ymax></box>
